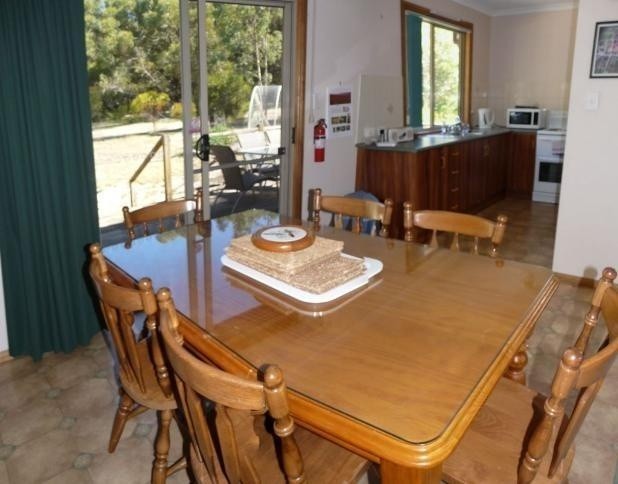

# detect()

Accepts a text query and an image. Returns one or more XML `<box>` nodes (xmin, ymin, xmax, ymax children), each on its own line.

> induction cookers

<box><xmin>537</xmin><ymin>126</ymin><xmax>566</xmax><ymax>135</ymax></box>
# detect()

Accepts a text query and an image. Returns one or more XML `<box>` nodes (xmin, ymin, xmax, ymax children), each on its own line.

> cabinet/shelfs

<box><xmin>353</xmin><ymin>122</ymin><xmax>539</xmax><ymax>244</ymax></box>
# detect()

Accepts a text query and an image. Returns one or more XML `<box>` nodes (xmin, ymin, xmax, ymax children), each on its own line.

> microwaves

<box><xmin>507</xmin><ymin>108</ymin><xmax>546</xmax><ymax>129</ymax></box>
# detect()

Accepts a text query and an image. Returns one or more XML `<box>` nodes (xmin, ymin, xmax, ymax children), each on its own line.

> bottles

<box><xmin>379</xmin><ymin>129</ymin><xmax>393</xmax><ymax>141</ymax></box>
<box><xmin>438</xmin><ymin>116</ymin><xmax>470</xmax><ymax>135</ymax></box>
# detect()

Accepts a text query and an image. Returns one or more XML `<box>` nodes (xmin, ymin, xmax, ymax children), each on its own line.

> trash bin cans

<box><xmin>340</xmin><ymin>191</ymin><xmax>380</xmax><ymax>235</ymax></box>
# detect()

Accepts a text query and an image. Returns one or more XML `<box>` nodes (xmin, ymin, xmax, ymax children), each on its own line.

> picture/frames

<box><xmin>588</xmin><ymin>21</ymin><xmax>617</xmax><ymax>80</ymax></box>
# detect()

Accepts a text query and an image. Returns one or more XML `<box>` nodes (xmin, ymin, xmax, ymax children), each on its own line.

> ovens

<box><xmin>533</xmin><ymin>135</ymin><xmax>566</xmax><ymax>205</ymax></box>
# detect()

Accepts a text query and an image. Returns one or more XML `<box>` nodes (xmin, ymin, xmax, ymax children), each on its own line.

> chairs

<box><xmin>313</xmin><ymin>187</ymin><xmax>393</xmax><ymax>238</ymax></box>
<box><xmin>402</xmin><ymin>200</ymin><xmax>506</xmax><ymax>258</ymax></box>
<box><xmin>205</xmin><ymin>125</ymin><xmax>285</xmax><ymax>216</ymax></box>
<box><xmin>89</xmin><ymin>242</ymin><xmax>213</xmax><ymax>483</ymax></box>
<box><xmin>122</xmin><ymin>193</ymin><xmax>202</xmax><ymax>241</ymax></box>
<box><xmin>432</xmin><ymin>266</ymin><xmax>616</xmax><ymax>484</ymax></box>
<box><xmin>151</xmin><ymin>287</ymin><xmax>375</xmax><ymax>484</ymax></box>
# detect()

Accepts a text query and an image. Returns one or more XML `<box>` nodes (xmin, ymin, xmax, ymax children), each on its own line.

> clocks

<box><xmin>251</xmin><ymin>225</ymin><xmax>315</xmax><ymax>252</ymax></box>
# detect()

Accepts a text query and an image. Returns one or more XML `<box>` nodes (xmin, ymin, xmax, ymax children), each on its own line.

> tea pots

<box><xmin>478</xmin><ymin>106</ymin><xmax>495</xmax><ymax>129</ymax></box>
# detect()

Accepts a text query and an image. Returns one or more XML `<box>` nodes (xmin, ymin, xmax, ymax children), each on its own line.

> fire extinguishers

<box><xmin>313</xmin><ymin>118</ymin><xmax>327</xmax><ymax>162</ymax></box>
